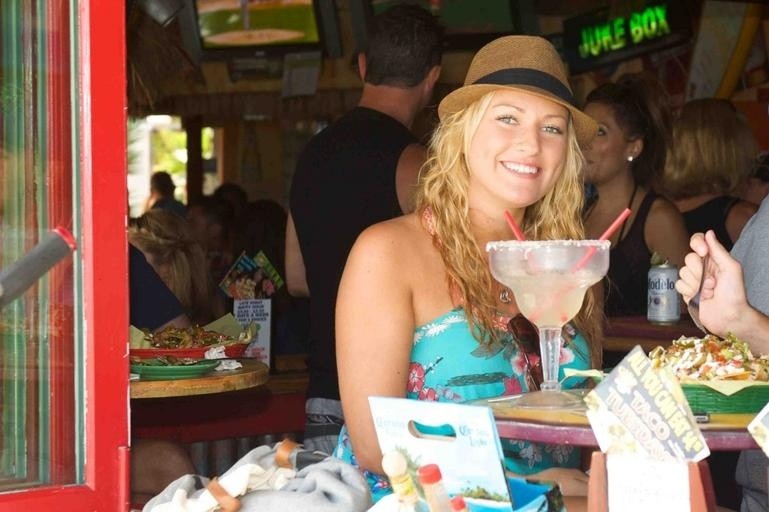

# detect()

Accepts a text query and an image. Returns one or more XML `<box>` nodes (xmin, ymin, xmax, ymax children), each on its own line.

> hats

<box><xmin>437</xmin><ymin>33</ymin><xmax>599</xmax><ymax>144</ymax></box>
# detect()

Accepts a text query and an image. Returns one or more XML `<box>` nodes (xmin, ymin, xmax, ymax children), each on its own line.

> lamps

<box><xmin>140</xmin><ymin>0</ymin><xmax>187</xmax><ymax>28</ymax></box>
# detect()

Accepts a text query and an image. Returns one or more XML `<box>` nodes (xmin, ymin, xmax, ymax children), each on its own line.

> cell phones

<box><xmin>689</xmin><ymin>406</ymin><xmax>711</xmax><ymax>421</ymax></box>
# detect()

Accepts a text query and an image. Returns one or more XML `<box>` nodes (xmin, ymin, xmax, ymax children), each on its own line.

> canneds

<box><xmin>646</xmin><ymin>259</ymin><xmax>682</xmax><ymax>327</ymax></box>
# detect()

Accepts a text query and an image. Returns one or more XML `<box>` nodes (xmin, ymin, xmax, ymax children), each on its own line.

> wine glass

<box><xmin>486</xmin><ymin>238</ymin><xmax>613</xmax><ymax>409</ymax></box>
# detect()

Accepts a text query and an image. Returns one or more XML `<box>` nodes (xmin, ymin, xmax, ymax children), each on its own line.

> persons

<box><xmin>580</xmin><ymin>82</ymin><xmax>760</xmax><ymax>315</ymax></box>
<box><xmin>127</xmin><ymin>173</ymin><xmax>288</xmax><ymax>502</ymax></box>
<box><xmin>283</xmin><ymin>4</ymin><xmax>444</xmax><ymax>427</ymax></box>
<box><xmin>333</xmin><ymin>38</ymin><xmax>606</xmax><ymax>512</ymax></box>
<box><xmin>673</xmin><ymin>195</ymin><xmax>769</xmax><ymax>512</ymax></box>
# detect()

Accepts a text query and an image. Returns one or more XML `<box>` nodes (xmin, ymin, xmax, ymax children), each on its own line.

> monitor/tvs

<box><xmin>177</xmin><ymin>1</ymin><xmax>343</xmax><ymax>62</ymax></box>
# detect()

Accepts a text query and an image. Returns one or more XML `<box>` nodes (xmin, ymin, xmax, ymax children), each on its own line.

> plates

<box><xmin>589</xmin><ymin>367</ymin><xmax>769</xmax><ymax>417</ymax></box>
<box><xmin>131</xmin><ymin>343</ymin><xmax>252</xmax><ymax>380</ymax></box>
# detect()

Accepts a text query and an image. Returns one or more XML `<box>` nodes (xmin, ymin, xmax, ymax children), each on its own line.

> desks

<box><xmin>125</xmin><ymin>280</ymin><xmax>769</xmax><ymax>512</ymax></box>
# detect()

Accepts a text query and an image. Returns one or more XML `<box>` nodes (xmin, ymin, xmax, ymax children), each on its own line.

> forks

<box><xmin>156</xmin><ymin>356</ymin><xmax>256</xmax><ymax>363</ymax></box>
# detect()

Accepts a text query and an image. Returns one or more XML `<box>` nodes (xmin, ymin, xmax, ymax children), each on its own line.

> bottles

<box><xmin>381</xmin><ymin>450</ymin><xmax>470</xmax><ymax>512</ymax></box>
